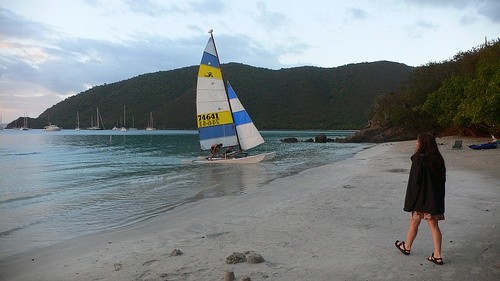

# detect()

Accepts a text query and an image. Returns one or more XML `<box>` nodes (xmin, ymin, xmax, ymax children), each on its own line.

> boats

<box><xmin>43</xmin><ymin>125</ymin><xmax>62</xmax><ymax>131</ymax></box>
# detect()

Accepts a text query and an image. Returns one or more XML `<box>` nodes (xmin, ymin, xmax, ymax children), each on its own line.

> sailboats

<box><xmin>87</xmin><ymin>108</ymin><xmax>104</xmax><ymax>129</ymax></box>
<box><xmin>18</xmin><ymin>111</ymin><xmax>29</xmax><ymax>130</ymax></box>
<box><xmin>74</xmin><ymin>110</ymin><xmax>80</xmax><ymax>130</ymax></box>
<box><xmin>111</xmin><ymin>105</ymin><xmax>166</xmax><ymax>131</ymax></box>
<box><xmin>189</xmin><ymin>28</ymin><xmax>276</xmax><ymax>164</ymax></box>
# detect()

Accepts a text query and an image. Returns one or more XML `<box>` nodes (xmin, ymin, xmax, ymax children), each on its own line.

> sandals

<box><xmin>395</xmin><ymin>240</ymin><xmax>410</xmax><ymax>256</ymax></box>
<box><xmin>426</xmin><ymin>252</ymin><xmax>444</xmax><ymax>265</ymax></box>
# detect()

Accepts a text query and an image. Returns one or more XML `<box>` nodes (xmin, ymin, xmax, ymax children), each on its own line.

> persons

<box><xmin>395</xmin><ymin>133</ymin><xmax>446</xmax><ymax>265</ymax></box>
<box><xmin>223</xmin><ymin>146</ymin><xmax>233</xmax><ymax>160</ymax></box>
<box><xmin>209</xmin><ymin>143</ymin><xmax>223</xmax><ymax>161</ymax></box>
